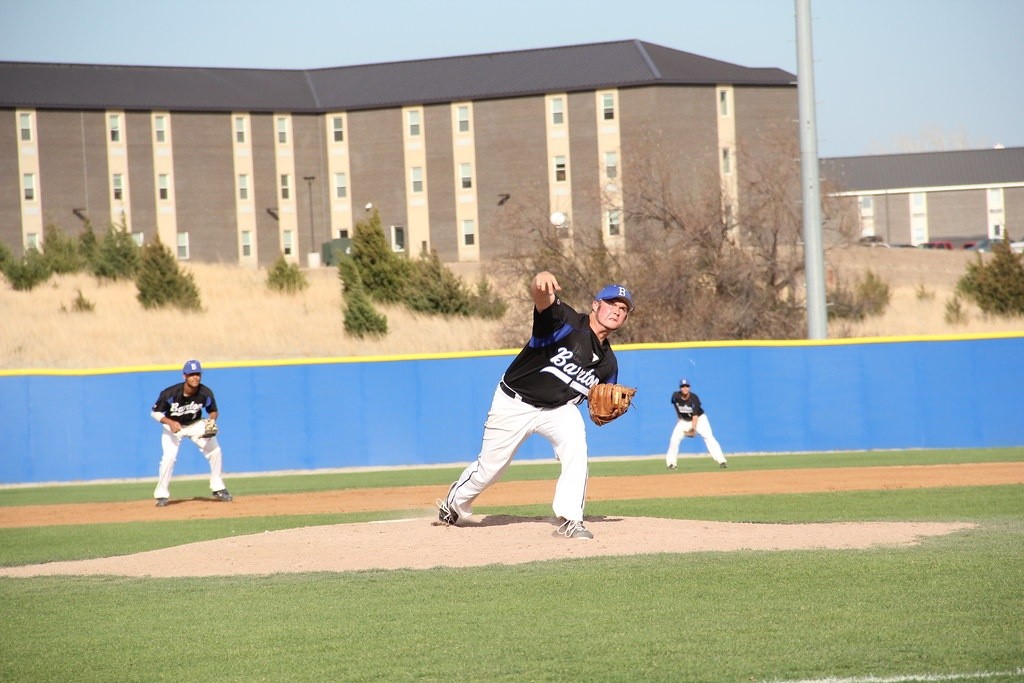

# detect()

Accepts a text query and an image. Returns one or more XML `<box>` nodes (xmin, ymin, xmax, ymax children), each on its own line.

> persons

<box><xmin>149</xmin><ymin>361</ymin><xmax>233</xmax><ymax>506</ymax></box>
<box><xmin>666</xmin><ymin>378</ymin><xmax>726</xmax><ymax>469</ymax></box>
<box><xmin>439</xmin><ymin>271</ymin><xmax>635</xmax><ymax>540</ymax></box>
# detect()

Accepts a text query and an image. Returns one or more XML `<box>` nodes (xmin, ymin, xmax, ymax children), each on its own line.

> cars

<box><xmin>856</xmin><ymin>233</ymin><xmax>1024</xmax><ymax>256</ymax></box>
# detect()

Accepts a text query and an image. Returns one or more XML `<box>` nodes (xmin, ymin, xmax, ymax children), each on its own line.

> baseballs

<box><xmin>549</xmin><ymin>211</ymin><xmax>566</xmax><ymax>226</ymax></box>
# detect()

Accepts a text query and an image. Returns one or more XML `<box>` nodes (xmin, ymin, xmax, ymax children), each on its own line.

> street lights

<box><xmin>302</xmin><ymin>175</ymin><xmax>317</xmax><ymax>253</ymax></box>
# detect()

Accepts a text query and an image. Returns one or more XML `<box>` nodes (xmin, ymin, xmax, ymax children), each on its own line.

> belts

<box><xmin>499</xmin><ymin>382</ymin><xmax>539</xmax><ymax>407</ymax></box>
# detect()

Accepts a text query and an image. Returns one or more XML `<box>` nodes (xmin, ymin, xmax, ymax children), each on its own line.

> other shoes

<box><xmin>668</xmin><ymin>465</ymin><xmax>676</xmax><ymax>470</ymax></box>
<box><xmin>720</xmin><ymin>462</ymin><xmax>727</xmax><ymax>467</ymax></box>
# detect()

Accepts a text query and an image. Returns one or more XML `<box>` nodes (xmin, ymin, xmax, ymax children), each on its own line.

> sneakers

<box><xmin>156</xmin><ymin>499</ymin><xmax>167</xmax><ymax>506</ymax></box>
<box><xmin>434</xmin><ymin>481</ymin><xmax>459</xmax><ymax>525</ymax></box>
<box><xmin>213</xmin><ymin>489</ymin><xmax>233</xmax><ymax>501</ymax></box>
<box><xmin>556</xmin><ymin>519</ymin><xmax>595</xmax><ymax>539</ymax></box>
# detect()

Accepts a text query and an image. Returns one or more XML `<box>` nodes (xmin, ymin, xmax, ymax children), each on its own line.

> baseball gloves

<box><xmin>198</xmin><ymin>418</ymin><xmax>218</xmax><ymax>439</ymax></box>
<box><xmin>683</xmin><ymin>428</ymin><xmax>697</xmax><ymax>438</ymax></box>
<box><xmin>587</xmin><ymin>383</ymin><xmax>637</xmax><ymax>426</ymax></box>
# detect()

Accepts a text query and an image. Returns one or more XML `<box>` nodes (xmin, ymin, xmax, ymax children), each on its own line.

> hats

<box><xmin>183</xmin><ymin>360</ymin><xmax>204</xmax><ymax>373</ymax></box>
<box><xmin>595</xmin><ymin>285</ymin><xmax>635</xmax><ymax>312</ymax></box>
<box><xmin>679</xmin><ymin>378</ymin><xmax>690</xmax><ymax>385</ymax></box>
